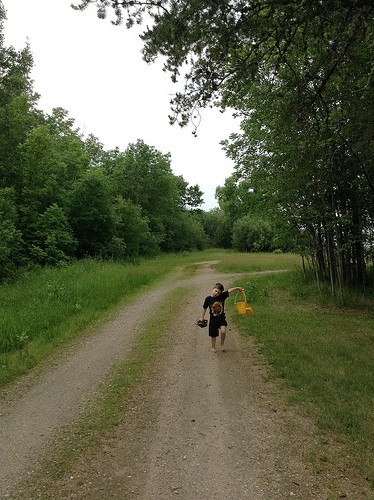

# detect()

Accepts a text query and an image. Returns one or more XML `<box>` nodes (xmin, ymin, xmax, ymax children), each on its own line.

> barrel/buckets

<box><xmin>234</xmin><ymin>290</ymin><xmax>247</xmax><ymax>316</ymax></box>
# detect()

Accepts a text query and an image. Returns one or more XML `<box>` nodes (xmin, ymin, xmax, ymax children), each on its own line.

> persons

<box><xmin>198</xmin><ymin>283</ymin><xmax>244</xmax><ymax>353</ymax></box>
